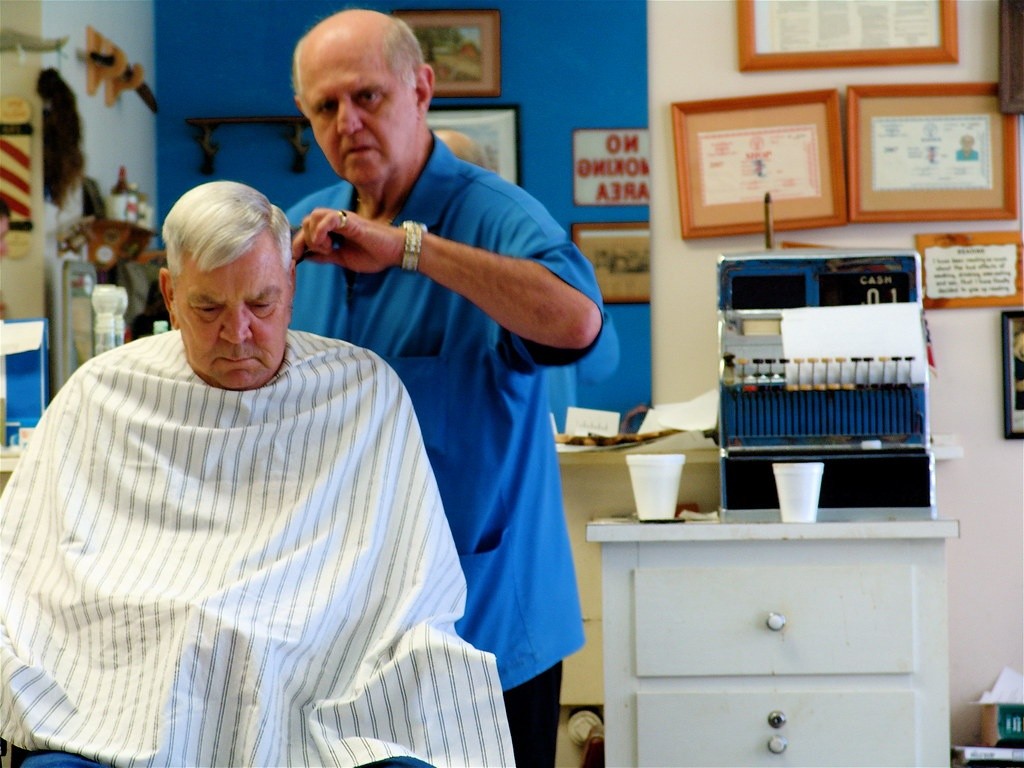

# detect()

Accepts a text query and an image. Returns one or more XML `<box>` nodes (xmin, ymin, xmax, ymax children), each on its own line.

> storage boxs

<box><xmin>964</xmin><ymin>700</ymin><xmax>1024</xmax><ymax>748</ymax></box>
<box><xmin>5</xmin><ymin>318</ymin><xmax>51</xmax><ymax>450</ymax></box>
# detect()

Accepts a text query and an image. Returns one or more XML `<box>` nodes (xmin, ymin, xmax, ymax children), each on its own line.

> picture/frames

<box><xmin>846</xmin><ymin>83</ymin><xmax>1020</xmax><ymax>225</ymax></box>
<box><xmin>425</xmin><ymin>103</ymin><xmax>524</xmax><ymax>192</ymax></box>
<box><xmin>570</xmin><ymin>221</ymin><xmax>651</xmax><ymax>305</ymax></box>
<box><xmin>736</xmin><ymin>0</ymin><xmax>960</xmax><ymax>73</ymax></box>
<box><xmin>669</xmin><ymin>88</ymin><xmax>847</xmax><ymax>242</ymax></box>
<box><xmin>998</xmin><ymin>0</ymin><xmax>1024</xmax><ymax>115</ymax></box>
<box><xmin>391</xmin><ymin>8</ymin><xmax>501</xmax><ymax>99</ymax></box>
<box><xmin>1002</xmin><ymin>311</ymin><xmax>1024</xmax><ymax>440</ymax></box>
<box><xmin>915</xmin><ymin>230</ymin><xmax>1024</xmax><ymax>309</ymax></box>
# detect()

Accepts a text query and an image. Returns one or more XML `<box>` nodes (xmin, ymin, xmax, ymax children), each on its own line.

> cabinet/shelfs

<box><xmin>584</xmin><ymin>515</ymin><xmax>961</xmax><ymax>768</ymax></box>
<box><xmin>557</xmin><ymin>449</ymin><xmax>721</xmax><ymax>705</ymax></box>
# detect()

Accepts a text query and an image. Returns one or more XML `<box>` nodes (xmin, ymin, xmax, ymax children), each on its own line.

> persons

<box><xmin>281</xmin><ymin>9</ymin><xmax>605</xmax><ymax>768</ymax></box>
<box><xmin>433</xmin><ymin>130</ymin><xmax>621</xmax><ymax>434</ymax></box>
<box><xmin>0</xmin><ymin>181</ymin><xmax>515</xmax><ymax>768</ymax></box>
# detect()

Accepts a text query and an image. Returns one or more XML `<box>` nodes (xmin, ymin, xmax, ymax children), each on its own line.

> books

<box><xmin>953</xmin><ymin>746</ymin><xmax>1024</xmax><ymax>766</ymax></box>
<box><xmin>979</xmin><ymin>703</ymin><xmax>1024</xmax><ymax>747</ymax></box>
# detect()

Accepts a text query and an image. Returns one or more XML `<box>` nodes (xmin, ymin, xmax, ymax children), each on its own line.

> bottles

<box><xmin>106</xmin><ymin>165</ymin><xmax>153</xmax><ymax>231</ymax></box>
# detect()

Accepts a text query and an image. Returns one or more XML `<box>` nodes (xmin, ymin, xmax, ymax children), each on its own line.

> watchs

<box><xmin>403</xmin><ymin>221</ymin><xmax>427</xmax><ymax>271</ymax></box>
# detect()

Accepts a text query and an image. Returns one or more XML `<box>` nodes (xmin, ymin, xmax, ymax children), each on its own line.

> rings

<box><xmin>338</xmin><ymin>211</ymin><xmax>347</xmax><ymax>228</ymax></box>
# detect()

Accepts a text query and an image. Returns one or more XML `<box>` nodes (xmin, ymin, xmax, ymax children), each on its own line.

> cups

<box><xmin>773</xmin><ymin>463</ymin><xmax>825</xmax><ymax>524</ymax></box>
<box><xmin>625</xmin><ymin>453</ymin><xmax>686</xmax><ymax>521</ymax></box>
<box><xmin>10</xmin><ymin>427</ymin><xmax>36</xmax><ymax>460</ymax></box>
